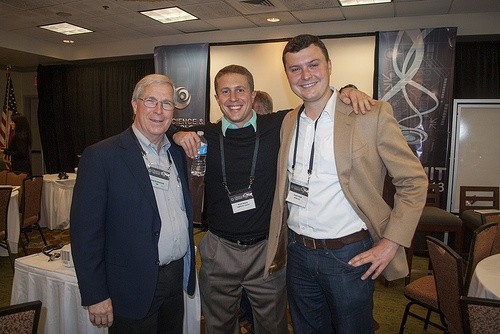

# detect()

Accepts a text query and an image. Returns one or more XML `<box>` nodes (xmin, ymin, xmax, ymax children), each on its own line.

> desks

<box><xmin>468</xmin><ymin>253</ymin><xmax>500</xmax><ymax>300</ymax></box>
<box><xmin>11</xmin><ymin>243</ymin><xmax>201</xmax><ymax>334</ymax></box>
<box><xmin>1</xmin><ymin>185</ymin><xmax>20</xmax><ymax>256</ymax></box>
<box><xmin>39</xmin><ymin>172</ymin><xmax>77</xmax><ymax>230</ymax></box>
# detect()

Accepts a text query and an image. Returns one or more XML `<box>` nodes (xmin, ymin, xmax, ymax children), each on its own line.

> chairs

<box><xmin>0</xmin><ymin>168</ymin><xmax>47</xmax><ymax>273</ymax></box>
<box><xmin>0</xmin><ymin>300</ymin><xmax>42</xmax><ymax>334</ymax></box>
<box><xmin>399</xmin><ymin>223</ymin><xmax>500</xmax><ymax>334</ymax></box>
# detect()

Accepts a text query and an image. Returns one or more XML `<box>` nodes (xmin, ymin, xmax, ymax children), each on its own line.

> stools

<box><xmin>405</xmin><ymin>185</ymin><xmax>462</xmax><ymax>283</ymax></box>
<box><xmin>460</xmin><ymin>186</ymin><xmax>499</xmax><ymax>223</ymax></box>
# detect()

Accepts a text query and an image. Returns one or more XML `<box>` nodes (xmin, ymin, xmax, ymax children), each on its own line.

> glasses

<box><xmin>139</xmin><ymin>96</ymin><xmax>176</xmax><ymax>111</ymax></box>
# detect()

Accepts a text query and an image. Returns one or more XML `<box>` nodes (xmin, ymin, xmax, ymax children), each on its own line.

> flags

<box><xmin>0</xmin><ymin>76</ymin><xmax>17</xmax><ymax>169</ymax></box>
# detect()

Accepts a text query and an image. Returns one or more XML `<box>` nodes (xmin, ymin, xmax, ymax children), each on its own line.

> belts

<box><xmin>288</xmin><ymin>228</ymin><xmax>371</xmax><ymax>251</ymax></box>
<box><xmin>206</xmin><ymin>225</ymin><xmax>269</xmax><ymax>246</ymax></box>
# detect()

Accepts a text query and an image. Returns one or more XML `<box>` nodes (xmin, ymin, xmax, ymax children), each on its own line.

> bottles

<box><xmin>191</xmin><ymin>131</ymin><xmax>208</xmax><ymax>177</ymax></box>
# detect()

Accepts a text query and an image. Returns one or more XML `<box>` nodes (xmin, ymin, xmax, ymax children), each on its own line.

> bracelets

<box><xmin>339</xmin><ymin>83</ymin><xmax>357</xmax><ymax>93</ymax></box>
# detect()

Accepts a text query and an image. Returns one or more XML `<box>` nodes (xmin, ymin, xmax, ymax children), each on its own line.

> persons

<box><xmin>166</xmin><ymin>64</ymin><xmax>376</xmax><ymax>334</ymax></box>
<box><xmin>65</xmin><ymin>74</ymin><xmax>194</xmax><ymax>334</ymax></box>
<box><xmin>0</xmin><ymin>111</ymin><xmax>35</xmax><ymax>176</ymax></box>
<box><xmin>236</xmin><ymin>89</ymin><xmax>273</xmax><ymax>334</ymax></box>
<box><xmin>262</xmin><ymin>34</ymin><xmax>427</xmax><ymax>334</ymax></box>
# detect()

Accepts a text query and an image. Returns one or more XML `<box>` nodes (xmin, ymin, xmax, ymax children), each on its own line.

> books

<box><xmin>474</xmin><ymin>209</ymin><xmax>500</xmax><ymax>217</ymax></box>
